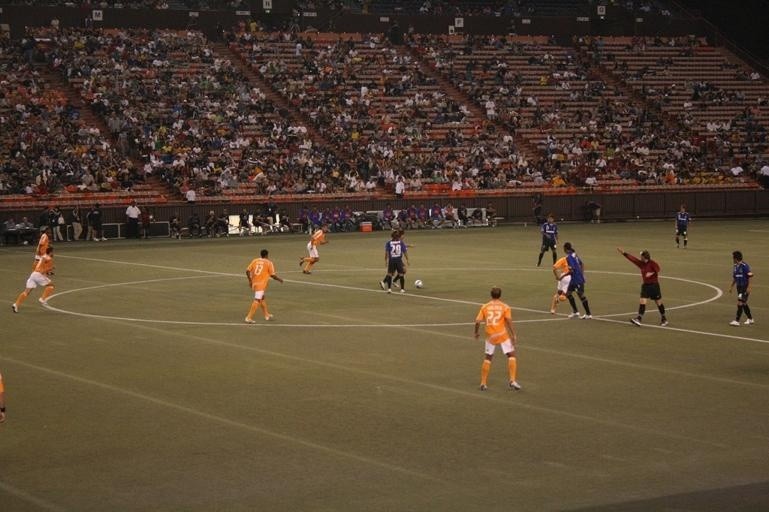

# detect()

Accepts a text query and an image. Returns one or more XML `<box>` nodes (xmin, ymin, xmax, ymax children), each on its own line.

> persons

<box><xmin>616</xmin><ymin>246</ymin><xmax>668</xmax><ymax>328</ymax></box>
<box><xmin>559</xmin><ymin>243</ymin><xmax>592</xmax><ymax>320</ymax></box>
<box><xmin>474</xmin><ymin>286</ymin><xmax>522</xmax><ymax>392</ymax></box>
<box><xmin>379</xmin><ymin>230</ymin><xmax>415</xmax><ymax>289</ymax></box>
<box><xmin>728</xmin><ymin>250</ymin><xmax>754</xmax><ymax>325</ymax></box>
<box><xmin>384</xmin><ymin>231</ymin><xmax>408</xmax><ymax>294</ymax></box>
<box><xmin>0</xmin><ymin>372</ymin><xmax>9</xmax><ymax>424</ymax></box>
<box><xmin>299</xmin><ymin>226</ymin><xmax>330</xmax><ymax>275</ymax></box>
<box><xmin>31</xmin><ymin>228</ymin><xmax>57</xmax><ymax>275</ymax></box>
<box><xmin>550</xmin><ymin>256</ymin><xmax>570</xmax><ymax>315</ymax></box>
<box><xmin>536</xmin><ymin>214</ymin><xmax>560</xmax><ymax>269</ymax></box>
<box><xmin>244</xmin><ymin>250</ymin><xmax>285</xmax><ymax>324</ymax></box>
<box><xmin>13</xmin><ymin>247</ymin><xmax>57</xmax><ymax>313</ymax></box>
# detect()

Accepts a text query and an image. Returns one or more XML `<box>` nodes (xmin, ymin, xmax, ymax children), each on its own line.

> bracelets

<box><xmin>1</xmin><ymin>406</ymin><xmax>6</xmax><ymax>412</ymax></box>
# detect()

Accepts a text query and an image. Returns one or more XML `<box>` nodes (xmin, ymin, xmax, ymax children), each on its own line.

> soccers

<box><xmin>415</xmin><ymin>279</ymin><xmax>423</xmax><ymax>288</ymax></box>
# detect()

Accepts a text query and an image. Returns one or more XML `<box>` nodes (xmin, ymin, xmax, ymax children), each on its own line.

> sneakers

<box><xmin>631</xmin><ymin>318</ymin><xmax>641</xmax><ymax>326</ymax></box>
<box><xmin>299</xmin><ymin>257</ymin><xmax>304</xmax><ymax>266</ymax></box>
<box><xmin>509</xmin><ymin>382</ymin><xmax>521</xmax><ymax>390</ymax></box>
<box><xmin>12</xmin><ymin>303</ymin><xmax>18</xmax><ymax>313</ymax></box>
<box><xmin>379</xmin><ymin>281</ymin><xmax>405</xmax><ymax>293</ymax></box>
<box><xmin>568</xmin><ymin>312</ymin><xmax>592</xmax><ymax>320</ymax></box>
<box><xmin>661</xmin><ymin>319</ymin><xmax>668</xmax><ymax>327</ymax></box>
<box><xmin>729</xmin><ymin>320</ymin><xmax>740</xmax><ymax>326</ymax></box>
<box><xmin>744</xmin><ymin>318</ymin><xmax>753</xmax><ymax>324</ymax></box>
<box><xmin>245</xmin><ymin>317</ymin><xmax>255</xmax><ymax>324</ymax></box>
<box><xmin>303</xmin><ymin>269</ymin><xmax>311</xmax><ymax>274</ymax></box>
